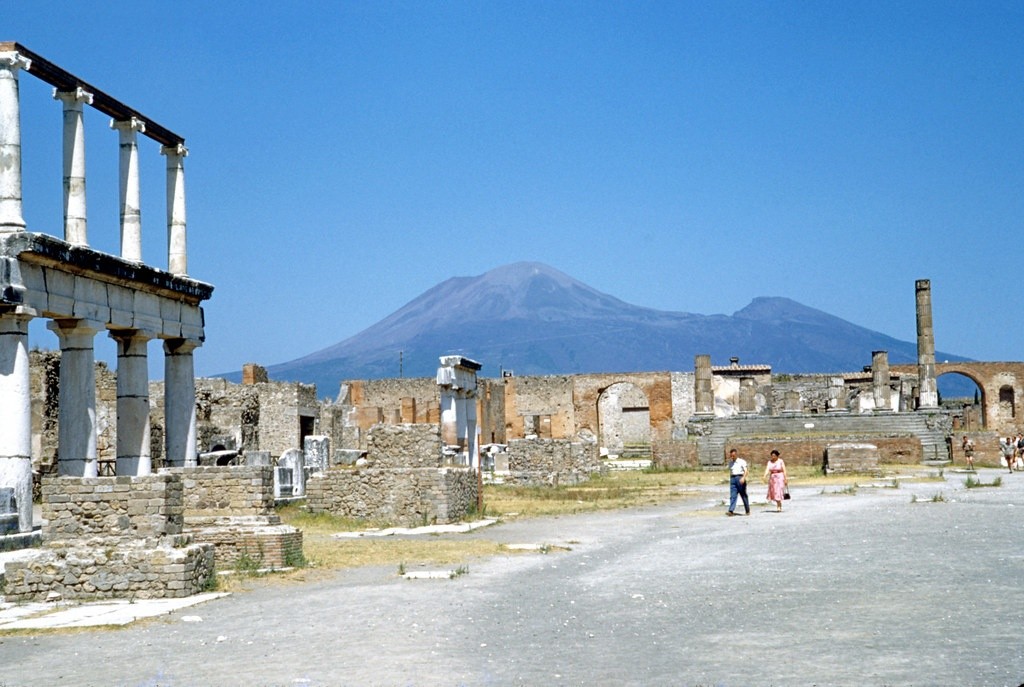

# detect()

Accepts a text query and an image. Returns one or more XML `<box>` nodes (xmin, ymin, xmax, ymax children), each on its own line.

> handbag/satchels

<box><xmin>784</xmin><ymin>485</ymin><xmax>790</xmax><ymax>499</ymax></box>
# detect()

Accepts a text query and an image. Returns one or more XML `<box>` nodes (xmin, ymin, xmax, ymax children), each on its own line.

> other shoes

<box><xmin>777</xmin><ymin>504</ymin><xmax>782</xmax><ymax>512</ymax></box>
<box><xmin>746</xmin><ymin>509</ymin><xmax>750</xmax><ymax>515</ymax></box>
<box><xmin>726</xmin><ymin>511</ymin><xmax>734</xmax><ymax>516</ymax></box>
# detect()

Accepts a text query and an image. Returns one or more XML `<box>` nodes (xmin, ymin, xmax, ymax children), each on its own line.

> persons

<box><xmin>962</xmin><ymin>435</ymin><xmax>977</xmax><ymax>469</ymax></box>
<box><xmin>999</xmin><ymin>432</ymin><xmax>1024</xmax><ymax>473</ymax></box>
<box><xmin>727</xmin><ymin>448</ymin><xmax>751</xmax><ymax>516</ymax></box>
<box><xmin>762</xmin><ymin>450</ymin><xmax>789</xmax><ymax>512</ymax></box>
